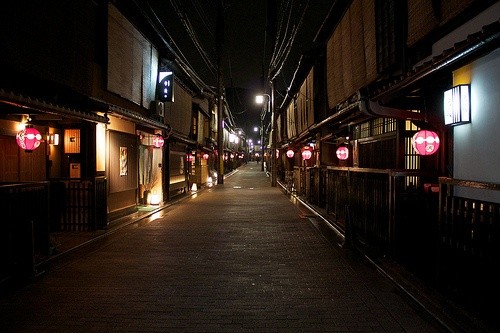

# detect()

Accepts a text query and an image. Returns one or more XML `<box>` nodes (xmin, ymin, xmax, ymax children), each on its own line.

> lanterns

<box><xmin>335</xmin><ymin>147</ymin><xmax>349</xmax><ymax>159</ymax></box>
<box><xmin>16</xmin><ymin>128</ymin><xmax>43</xmax><ymax>150</ymax></box>
<box><xmin>412</xmin><ymin>128</ymin><xmax>439</xmax><ymax>157</ymax></box>
<box><xmin>302</xmin><ymin>151</ymin><xmax>313</xmax><ymax>160</ymax></box>
<box><xmin>133</xmin><ymin>119</ymin><xmax>298</xmax><ymax>183</ymax></box>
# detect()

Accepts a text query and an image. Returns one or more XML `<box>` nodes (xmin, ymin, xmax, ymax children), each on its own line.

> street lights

<box><xmin>257</xmin><ymin>93</ymin><xmax>276</xmax><ymax>187</ymax></box>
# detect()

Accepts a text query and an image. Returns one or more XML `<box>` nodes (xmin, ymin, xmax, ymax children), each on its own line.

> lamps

<box><xmin>15</xmin><ymin>124</ymin><xmax>42</xmax><ymax>152</ymax></box>
<box><xmin>336</xmin><ymin>144</ymin><xmax>349</xmax><ymax>161</ymax></box>
<box><xmin>287</xmin><ymin>149</ymin><xmax>294</xmax><ymax>158</ymax></box>
<box><xmin>412</xmin><ymin>129</ymin><xmax>440</xmax><ymax>158</ymax></box>
<box><xmin>443</xmin><ymin>84</ymin><xmax>471</xmax><ymax>128</ymax></box>
<box><xmin>302</xmin><ymin>149</ymin><xmax>312</xmax><ymax>160</ymax></box>
